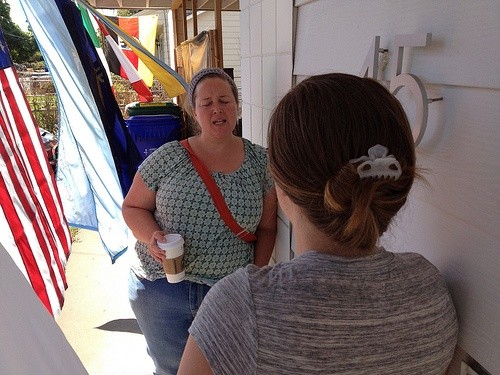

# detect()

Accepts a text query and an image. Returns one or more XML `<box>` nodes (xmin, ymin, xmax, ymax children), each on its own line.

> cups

<box><xmin>157</xmin><ymin>234</ymin><xmax>185</xmax><ymax>284</ymax></box>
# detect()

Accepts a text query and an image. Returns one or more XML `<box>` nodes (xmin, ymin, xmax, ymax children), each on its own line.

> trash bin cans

<box><xmin>128</xmin><ymin>102</ymin><xmax>181</xmax><ymax>116</ymax></box>
<box><xmin>125</xmin><ymin>115</ymin><xmax>180</xmax><ymax>159</ymax></box>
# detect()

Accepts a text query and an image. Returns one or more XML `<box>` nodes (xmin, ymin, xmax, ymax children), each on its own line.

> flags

<box><xmin>75</xmin><ymin>0</ymin><xmax>116</xmax><ymax>100</ymax></box>
<box><xmin>19</xmin><ymin>0</ymin><xmax>145</xmax><ymax>264</ymax></box>
<box><xmin>98</xmin><ymin>14</ymin><xmax>159</xmax><ymax>87</ymax></box>
<box><xmin>80</xmin><ymin>0</ymin><xmax>190</xmax><ymax>99</ymax></box>
<box><xmin>89</xmin><ymin>10</ymin><xmax>153</xmax><ymax>103</ymax></box>
<box><xmin>0</xmin><ymin>23</ymin><xmax>72</xmax><ymax>320</ymax></box>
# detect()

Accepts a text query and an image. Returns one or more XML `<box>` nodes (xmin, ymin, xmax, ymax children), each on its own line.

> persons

<box><xmin>122</xmin><ymin>68</ymin><xmax>278</xmax><ymax>375</ymax></box>
<box><xmin>177</xmin><ymin>73</ymin><xmax>459</xmax><ymax>375</ymax></box>
